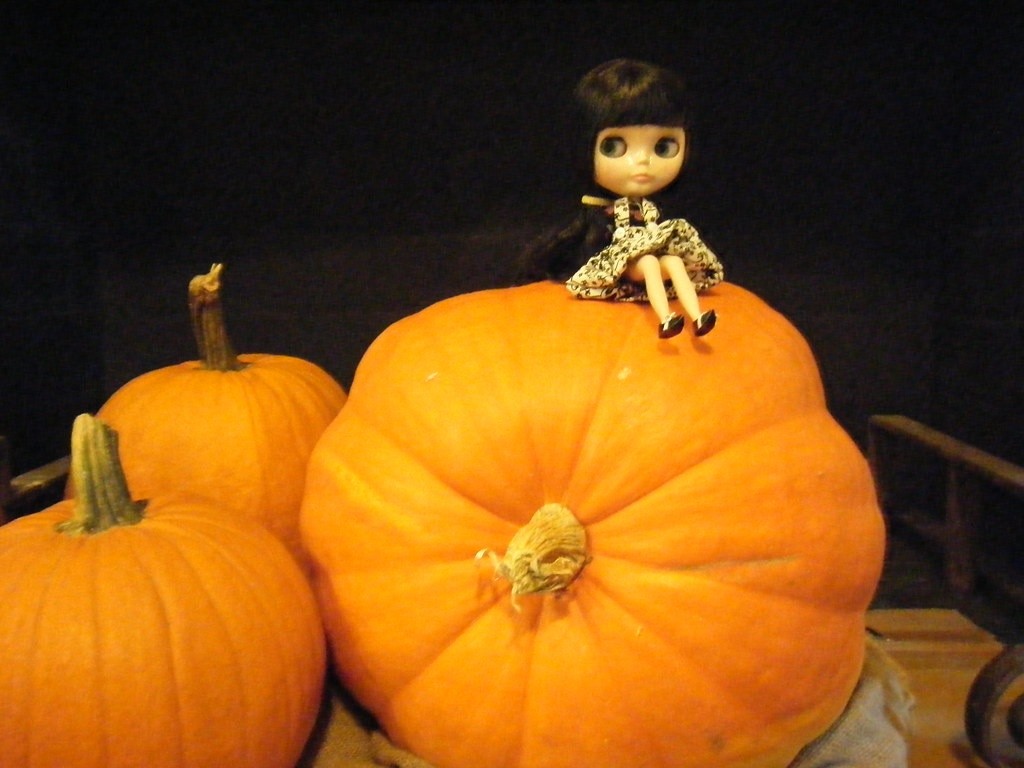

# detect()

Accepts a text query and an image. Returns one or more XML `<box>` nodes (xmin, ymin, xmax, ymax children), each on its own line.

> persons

<box><xmin>567</xmin><ymin>55</ymin><xmax>725</xmax><ymax>339</ymax></box>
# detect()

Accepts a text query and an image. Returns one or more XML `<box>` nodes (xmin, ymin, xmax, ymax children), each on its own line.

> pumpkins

<box><xmin>298</xmin><ymin>275</ymin><xmax>884</xmax><ymax>767</ymax></box>
<box><xmin>94</xmin><ymin>268</ymin><xmax>351</xmax><ymax>545</ymax></box>
<box><xmin>0</xmin><ymin>412</ymin><xmax>327</xmax><ymax>768</ymax></box>
